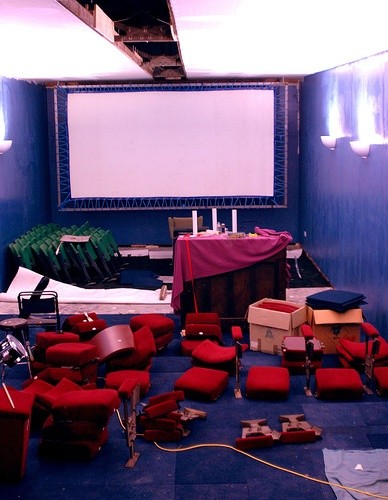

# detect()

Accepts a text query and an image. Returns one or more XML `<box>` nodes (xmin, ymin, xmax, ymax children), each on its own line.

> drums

<box><xmin>0</xmin><ymin>318</ymin><xmax>29</xmax><ymax>349</ymax></box>
<box><xmin>0</xmin><ymin>334</ymin><xmax>29</xmax><ymax>368</ymax></box>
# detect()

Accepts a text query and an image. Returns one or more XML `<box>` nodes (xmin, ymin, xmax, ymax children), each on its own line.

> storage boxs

<box><xmin>243</xmin><ymin>298</ymin><xmax>306</xmax><ymax>358</ymax></box>
<box><xmin>307</xmin><ymin>304</ymin><xmax>364</xmax><ymax>356</ymax></box>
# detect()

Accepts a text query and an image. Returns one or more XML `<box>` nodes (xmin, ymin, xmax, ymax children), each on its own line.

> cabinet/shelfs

<box><xmin>171</xmin><ymin>231</ymin><xmax>294</xmax><ymax>331</ymax></box>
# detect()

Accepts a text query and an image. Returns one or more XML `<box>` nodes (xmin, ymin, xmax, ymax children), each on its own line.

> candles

<box><xmin>232</xmin><ymin>209</ymin><xmax>237</xmax><ymax>233</ymax></box>
<box><xmin>212</xmin><ymin>207</ymin><xmax>218</xmax><ymax>231</ymax></box>
<box><xmin>192</xmin><ymin>210</ymin><xmax>198</xmax><ymax>235</ymax></box>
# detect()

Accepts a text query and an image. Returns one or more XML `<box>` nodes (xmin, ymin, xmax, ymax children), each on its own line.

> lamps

<box><xmin>350</xmin><ymin>140</ymin><xmax>371</xmax><ymax>159</ymax></box>
<box><xmin>319</xmin><ymin>135</ymin><xmax>338</xmax><ymax>153</ymax></box>
<box><xmin>0</xmin><ymin>139</ymin><xmax>13</xmax><ymax>155</ymax></box>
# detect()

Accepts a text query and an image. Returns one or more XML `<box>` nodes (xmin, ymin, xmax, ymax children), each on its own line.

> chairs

<box><xmin>168</xmin><ymin>216</ymin><xmax>204</xmax><ymax>239</ymax></box>
<box><xmin>8</xmin><ymin>221</ymin><xmax>129</xmax><ymax>286</ymax></box>
<box><xmin>17</xmin><ymin>290</ymin><xmax>63</xmax><ymax>333</ymax></box>
